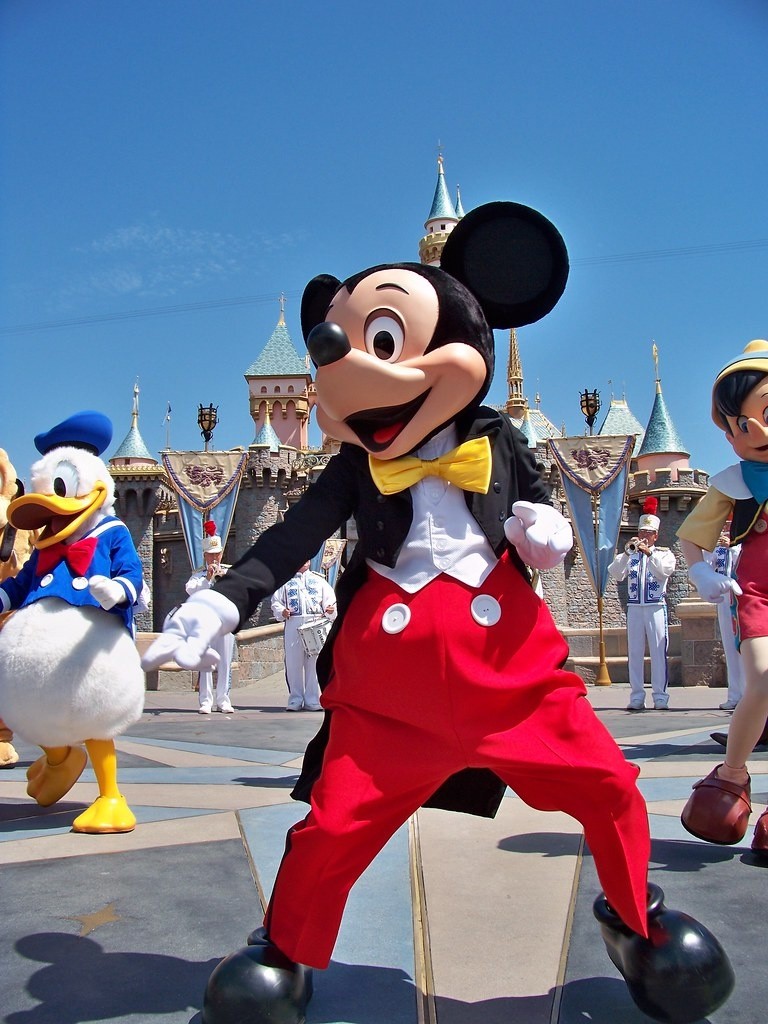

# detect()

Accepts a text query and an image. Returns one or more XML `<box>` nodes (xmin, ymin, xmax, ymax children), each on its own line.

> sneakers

<box><xmin>216</xmin><ymin>704</ymin><xmax>235</xmax><ymax>713</ymax></box>
<box><xmin>285</xmin><ymin>705</ymin><xmax>303</xmax><ymax>710</ymax></box>
<box><xmin>303</xmin><ymin>704</ymin><xmax>323</xmax><ymax>711</ymax></box>
<box><xmin>718</xmin><ymin>700</ymin><xmax>737</xmax><ymax>709</ymax></box>
<box><xmin>626</xmin><ymin>701</ymin><xmax>645</xmax><ymax>709</ymax></box>
<box><xmin>653</xmin><ymin>699</ymin><xmax>670</xmax><ymax>710</ymax></box>
<box><xmin>198</xmin><ymin>706</ymin><xmax>212</xmax><ymax>714</ymax></box>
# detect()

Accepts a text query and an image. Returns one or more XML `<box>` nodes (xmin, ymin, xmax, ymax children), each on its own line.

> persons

<box><xmin>608</xmin><ymin>514</ymin><xmax>676</xmax><ymax>710</ymax></box>
<box><xmin>270</xmin><ymin>560</ymin><xmax>337</xmax><ymax>711</ymax></box>
<box><xmin>701</xmin><ymin>521</ymin><xmax>747</xmax><ymax>710</ymax></box>
<box><xmin>186</xmin><ymin>536</ymin><xmax>234</xmax><ymax>714</ymax></box>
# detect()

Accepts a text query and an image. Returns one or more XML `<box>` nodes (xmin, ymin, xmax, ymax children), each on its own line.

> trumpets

<box><xmin>212</xmin><ymin>559</ymin><xmax>222</xmax><ymax>581</ymax></box>
<box><xmin>624</xmin><ymin>537</ymin><xmax>647</xmax><ymax>556</ymax></box>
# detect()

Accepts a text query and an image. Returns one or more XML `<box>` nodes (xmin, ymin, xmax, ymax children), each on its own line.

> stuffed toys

<box><xmin>0</xmin><ymin>410</ymin><xmax>146</xmax><ymax>833</ymax></box>
<box><xmin>0</xmin><ymin>448</ymin><xmax>41</xmax><ymax>768</ymax></box>
<box><xmin>143</xmin><ymin>201</ymin><xmax>737</xmax><ymax>1024</ymax></box>
<box><xmin>677</xmin><ymin>339</ymin><xmax>768</xmax><ymax>853</ymax></box>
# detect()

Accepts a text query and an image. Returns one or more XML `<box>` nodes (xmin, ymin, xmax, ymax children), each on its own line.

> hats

<box><xmin>202</xmin><ymin>520</ymin><xmax>222</xmax><ymax>554</ymax></box>
<box><xmin>638</xmin><ymin>514</ymin><xmax>661</xmax><ymax>531</ymax></box>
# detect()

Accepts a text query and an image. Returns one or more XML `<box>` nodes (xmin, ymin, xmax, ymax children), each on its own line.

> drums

<box><xmin>297</xmin><ymin>618</ymin><xmax>333</xmax><ymax>656</ymax></box>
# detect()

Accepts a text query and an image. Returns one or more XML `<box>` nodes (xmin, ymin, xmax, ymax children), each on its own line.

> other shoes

<box><xmin>709</xmin><ymin>732</ymin><xmax>730</xmax><ymax>747</ymax></box>
<box><xmin>751</xmin><ymin>744</ymin><xmax>768</xmax><ymax>751</ymax></box>
